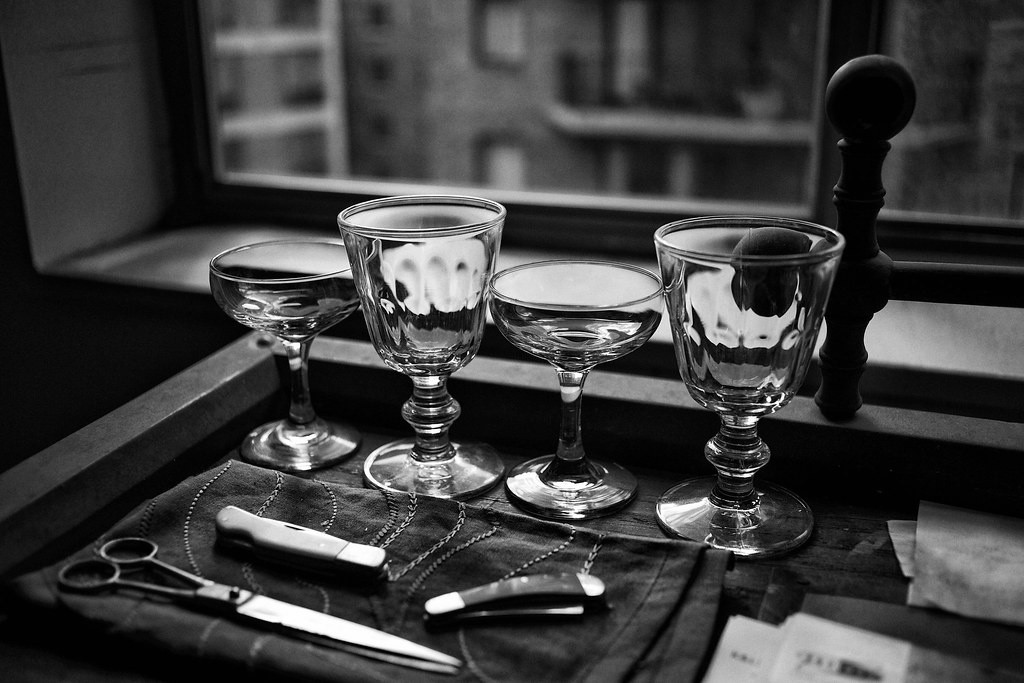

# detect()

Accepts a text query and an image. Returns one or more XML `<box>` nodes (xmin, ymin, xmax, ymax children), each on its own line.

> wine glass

<box><xmin>337</xmin><ymin>194</ymin><xmax>507</xmax><ymax>501</ymax></box>
<box><xmin>485</xmin><ymin>260</ymin><xmax>664</xmax><ymax>519</ymax></box>
<box><xmin>208</xmin><ymin>238</ymin><xmax>362</xmax><ymax>474</ymax></box>
<box><xmin>654</xmin><ymin>214</ymin><xmax>846</xmax><ymax>556</ymax></box>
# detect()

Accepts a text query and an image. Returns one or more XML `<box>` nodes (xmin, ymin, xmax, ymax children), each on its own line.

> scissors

<box><xmin>53</xmin><ymin>535</ymin><xmax>461</xmax><ymax>673</ymax></box>
